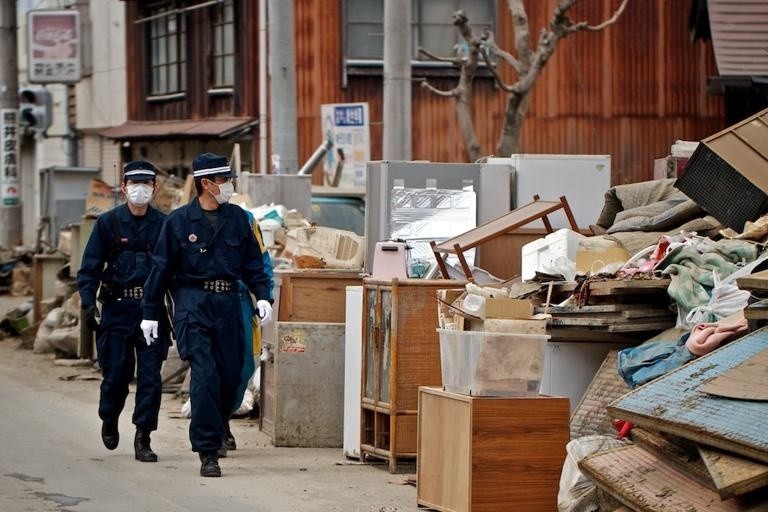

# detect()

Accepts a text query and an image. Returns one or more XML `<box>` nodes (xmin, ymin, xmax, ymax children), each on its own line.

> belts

<box><xmin>205</xmin><ymin>280</ymin><xmax>236</xmax><ymax>293</ymax></box>
<box><xmin>122</xmin><ymin>287</ymin><xmax>144</xmax><ymax>300</ymax></box>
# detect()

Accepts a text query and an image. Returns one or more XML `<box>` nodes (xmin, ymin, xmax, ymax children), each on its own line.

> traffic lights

<box><xmin>18</xmin><ymin>89</ymin><xmax>53</xmax><ymax>131</ymax></box>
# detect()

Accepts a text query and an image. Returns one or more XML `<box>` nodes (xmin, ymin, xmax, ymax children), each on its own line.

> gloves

<box><xmin>256</xmin><ymin>300</ymin><xmax>274</xmax><ymax>326</ymax></box>
<box><xmin>140</xmin><ymin>320</ymin><xmax>159</xmax><ymax>345</ymax></box>
<box><xmin>81</xmin><ymin>305</ymin><xmax>101</xmax><ymax>330</ymax></box>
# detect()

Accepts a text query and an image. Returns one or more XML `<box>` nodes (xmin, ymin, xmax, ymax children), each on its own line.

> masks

<box><xmin>204</xmin><ymin>180</ymin><xmax>234</xmax><ymax>205</ymax></box>
<box><xmin>124</xmin><ymin>184</ymin><xmax>154</xmax><ymax>207</ymax></box>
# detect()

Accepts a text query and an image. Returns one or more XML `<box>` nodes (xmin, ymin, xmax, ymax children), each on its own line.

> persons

<box><xmin>140</xmin><ymin>152</ymin><xmax>273</xmax><ymax>477</ymax></box>
<box><xmin>78</xmin><ymin>160</ymin><xmax>173</xmax><ymax>463</ymax></box>
<box><xmin>222</xmin><ymin>202</ymin><xmax>275</xmax><ymax>451</ymax></box>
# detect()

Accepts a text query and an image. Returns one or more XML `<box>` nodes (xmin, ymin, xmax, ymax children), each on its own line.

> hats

<box><xmin>192</xmin><ymin>152</ymin><xmax>238</xmax><ymax>178</ymax></box>
<box><xmin>124</xmin><ymin>161</ymin><xmax>157</xmax><ymax>181</ymax></box>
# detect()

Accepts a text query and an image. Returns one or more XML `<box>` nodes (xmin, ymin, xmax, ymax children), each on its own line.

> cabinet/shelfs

<box><xmin>360</xmin><ymin>277</ymin><xmax>472</xmax><ymax>474</ymax></box>
<box><xmin>258</xmin><ymin>321</ymin><xmax>345</xmax><ymax>449</ymax></box>
<box><xmin>431</xmin><ymin>193</ymin><xmax>583</xmax><ymax>303</ymax></box>
<box><xmin>417</xmin><ymin>385</ymin><xmax>570</xmax><ymax>512</ymax></box>
<box><xmin>673</xmin><ymin>106</ymin><xmax>768</xmax><ymax>233</ymax></box>
<box><xmin>270</xmin><ymin>270</ymin><xmax>368</xmax><ymax>323</ymax></box>
<box><xmin>34</xmin><ymin>253</ymin><xmax>66</xmax><ymax>325</ymax></box>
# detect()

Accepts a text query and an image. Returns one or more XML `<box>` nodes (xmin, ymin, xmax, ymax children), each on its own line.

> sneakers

<box><xmin>102</xmin><ymin>419</ymin><xmax>235</xmax><ymax>475</ymax></box>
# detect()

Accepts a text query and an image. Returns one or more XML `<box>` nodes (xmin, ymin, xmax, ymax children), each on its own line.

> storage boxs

<box><xmin>6</xmin><ymin>302</ymin><xmax>36</xmax><ymax>335</ymax></box>
<box><xmin>437</xmin><ymin>328</ymin><xmax>551</xmax><ymax>398</ymax></box>
